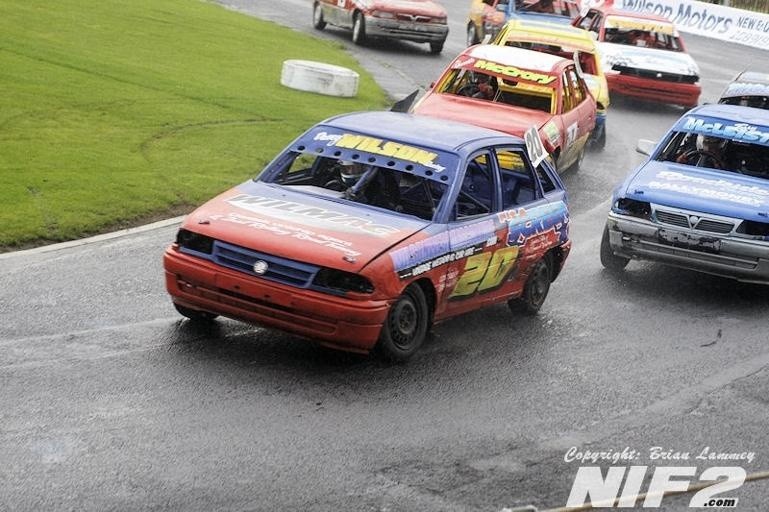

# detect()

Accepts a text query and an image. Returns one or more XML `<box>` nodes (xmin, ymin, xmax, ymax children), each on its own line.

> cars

<box><xmin>163</xmin><ymin>0</ymin><xmax>769</xmax><ymax>361</ymax></box>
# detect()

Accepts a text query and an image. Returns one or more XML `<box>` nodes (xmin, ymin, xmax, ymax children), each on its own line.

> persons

<box><xmin>467</xmin><ymin>71</ymin><xmax>502</xmax><ymax>103</ymax></box>
<box><xmin>675</xmin><ymin>133</ymin><xmax>731</xmax><ymax>169</ymax></box>
<box><xmin>321</xmin><ymin>159</ymin><xmax>369</xmax><ymax>202</ymax></box>
